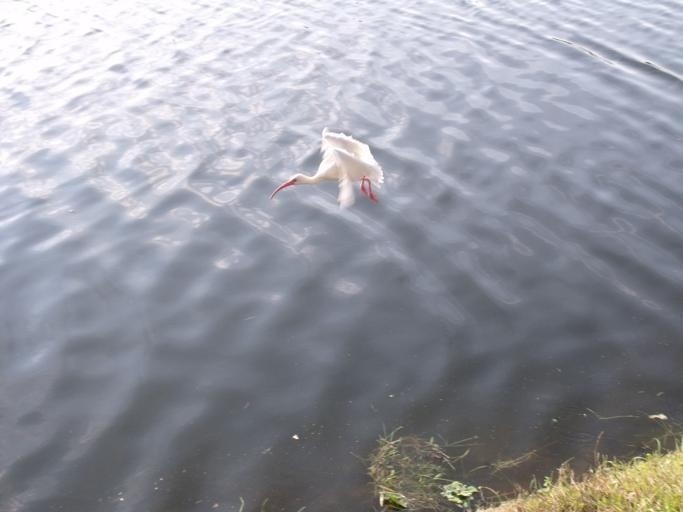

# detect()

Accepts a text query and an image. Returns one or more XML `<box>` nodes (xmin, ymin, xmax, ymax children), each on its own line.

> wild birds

<box><xmin>268</xmin><ymin>126</ymin><xmax>385</xmax><ymax>210</ymax></box>
